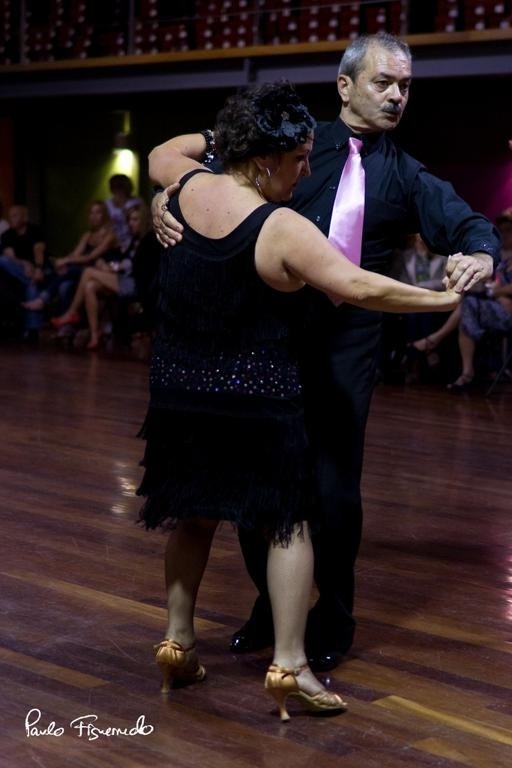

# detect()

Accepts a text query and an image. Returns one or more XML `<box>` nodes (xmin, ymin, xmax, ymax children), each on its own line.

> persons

<box><xmin>136</xmin><ymin>76</ymin><xmax>469</xmax><ymax>725</ymax></box>
<box><xmin>148</xmin><ymin>26</ymin><xmax>502</xmax><ymax>675</ymax></box>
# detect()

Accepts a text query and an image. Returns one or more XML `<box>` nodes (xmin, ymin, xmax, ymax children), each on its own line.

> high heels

<box><xmin>87</xmin><ymin>331</ymin><xmax>103</xmax><ymax>348</ymax></box>
<box><xmin>264</xmin><ymin>662</ymin><xmax>349</xmax><ymax>721</ymax></box>
<box><xmin>51</xmin><ymin>312</ymin><xmax>81</xmax><ymax>327</ymax></box>
<box><xmin>410</xmin><ymin>337</ymin><xmax>437</xmax><ymax>358</ymax></box>
<box><xmin>154</xmin><ymin>639</ymin><xmax>206</xmax><ymax>693</ymax></box>
<box><xmin>445</xmin><ymin>374</ymin><xmax>477</xmax><ymax>396</ymax></box>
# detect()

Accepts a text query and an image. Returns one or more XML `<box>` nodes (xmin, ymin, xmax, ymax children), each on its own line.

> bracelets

<box><xmin>200</xmin><ymin>124</ymin><xmax>217</xmax><ymax>166</ymax></box>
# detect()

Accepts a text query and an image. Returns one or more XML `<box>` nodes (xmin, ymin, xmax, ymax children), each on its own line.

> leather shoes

<box><xmin>308</xmin><ymin>644</ymin><xmax>342</xmax><ymax>672</ymax></box>
<box><xmin>229</xmin><ymin>619</ymin><xmax>271</xmax><ymax>651</ymax></box>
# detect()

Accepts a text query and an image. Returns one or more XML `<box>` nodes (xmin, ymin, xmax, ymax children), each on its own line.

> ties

<box><xmin>325</xmin><ymin>135</ymin><xmax>367</xmax><ymax>310</ymax></box>
